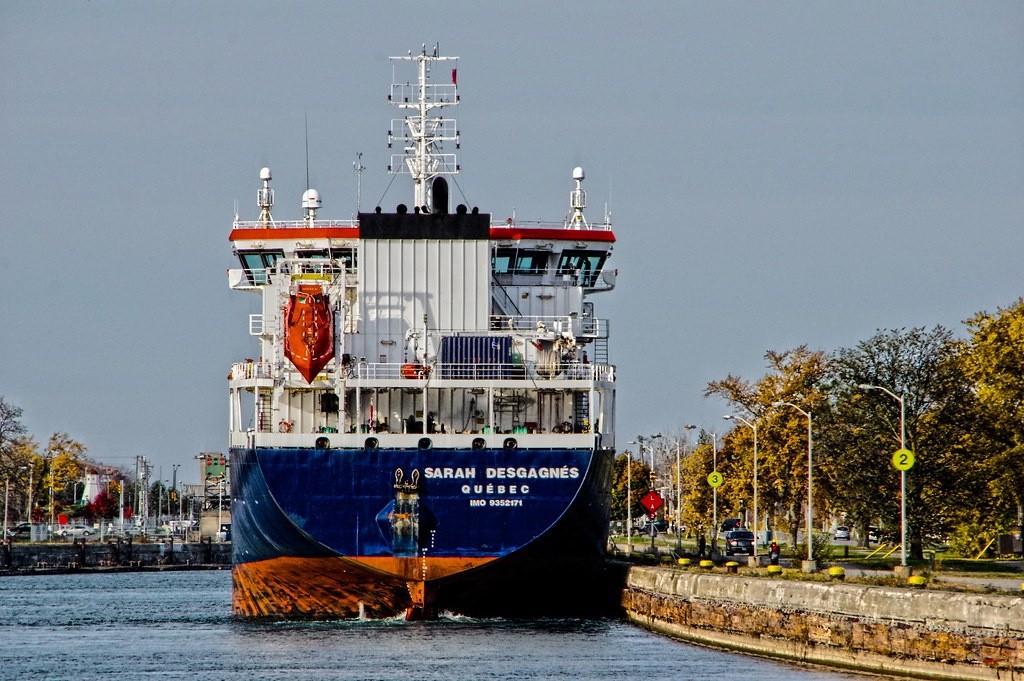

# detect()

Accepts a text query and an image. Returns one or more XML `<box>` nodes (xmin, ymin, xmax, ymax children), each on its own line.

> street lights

<box><xmin>652</xmin><ymin>432</ymin><xmax>685</xmax><ymax>556</ymax></box>
<box><xmin>683</xmin><ymin>424</ymin><xmax>722</xmax><ymax>562</ymax></box>
<box><xmin>627</xmin><ymin>439</ymin><xmax>659</xmax><ymax>555</ymax></box>
<box><xmin>722</xmin><ymin>415</ymin><xmax>762</xmax><ymax>568</ymax></box>
<box><xmin>859</xmin><ymin>385</ymin><xmax>913</xmax><ymax>579</ymax></box>
<box><xmin>771</xmin><ymin>401</ymin><xmax>817</xmax><ymax>575</ymax></box>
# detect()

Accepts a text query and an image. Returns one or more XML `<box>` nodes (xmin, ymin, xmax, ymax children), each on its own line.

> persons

<box><xmin>697</xmin><ymin>535</ymin><xmax>707</xmax><ymax>556</ymax></box>
<box><xmin>6</xmin><ymin>527</ymin><xmax>13</xmax><ymax>545</ymax></box>
<box><xmin>107</xmin><ymin>524</ymin><xmax>114</xmax><ymax>534</ymax></box>
<box><xmin>216</xmin><ymin>529</ymin><xmax>221</xmax><ymax>542</ymax></box>
<box><xmin>768</xmin><ymin>542</ymin><xmax>780</xmax><ymax>566</ymax></box>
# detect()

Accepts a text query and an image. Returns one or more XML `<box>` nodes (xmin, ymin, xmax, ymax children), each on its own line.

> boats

<box><xmin>280</xmin><ymin>282</ymin><xmax>342</xmax><ymax>388</ymax></box>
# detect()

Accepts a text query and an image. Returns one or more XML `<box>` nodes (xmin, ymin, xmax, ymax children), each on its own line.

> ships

<box><xmin>221</xmin><ymin>38</ymin><xmax>614</xmax><ymax>623</ymax></box>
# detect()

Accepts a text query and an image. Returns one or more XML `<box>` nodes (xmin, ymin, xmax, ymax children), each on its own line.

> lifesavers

<box><xmin>562</xmin><ymin>422</ymin><xmax>573</xmax><ymax>433</ymax></box>
<box><xmin>278</xmin><ymin>422</ymin><xmax>291</xmax><ymax>433</ymax></box>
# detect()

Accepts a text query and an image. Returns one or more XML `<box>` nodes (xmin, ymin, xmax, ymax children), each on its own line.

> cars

<box><xmin>834</xmin><ymin>526</ymin><xmax>850</xmax><ymax>539</ymax></box>
<box><xmin>9</xmin><ymin>523</ymin><xmax>31</xmax><ymax>534</ymax></box>
<box><xmin>58</xmin><ymin>524</ymin><xmax>96</xmax><ymax>537</ymax></box>
<box><xmin>721</xmin><ymin>518</ymin><xmax>743</xmax><ymax>531</ymax></box>
<box><xmin>650</xmin><ymin>520</ymin><xmax>672</xmax><ymax>532</ymax></box>
<box><xmin>125</xmin><ymin>527</ymin><xmax>145</xmax><ymax>535</ymax></box>
<box><xmin>868</xmin><ymin>526</ymin><xmax>880</xmax><ymax>543</ymax></box>
<box><xmin>724</xmin><ymin>530</ymin><xmax>754</xmax><ymax>556</ymax></box>
